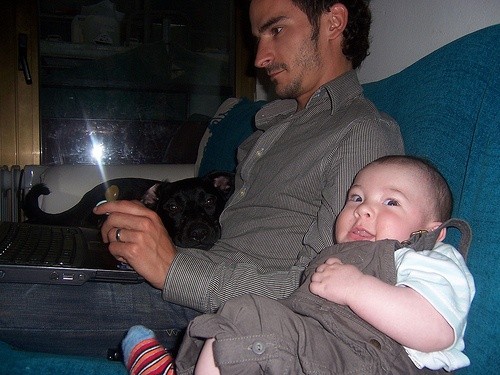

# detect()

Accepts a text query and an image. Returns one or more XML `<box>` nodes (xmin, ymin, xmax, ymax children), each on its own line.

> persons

<box><xmin>0</xmin><ymin>1</ymin><xmax>406</xmax><ymax>359</ymax></box>
<box><xmin>121</xmin><ymin>153</ymin><xmax>477</xmax><ymax>375</ymax></box>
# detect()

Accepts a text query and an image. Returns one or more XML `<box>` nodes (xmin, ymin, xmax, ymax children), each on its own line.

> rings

<box><xmin>115</xmin><ymin>227</ymin><xmax>126</xmax><ymax>243</ymax></box>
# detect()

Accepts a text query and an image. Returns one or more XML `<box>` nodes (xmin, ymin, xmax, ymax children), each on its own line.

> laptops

<box><xmin>0</xmin><ymin>221</ymin><xmax>144</xmax><ymax>286</ymax></box>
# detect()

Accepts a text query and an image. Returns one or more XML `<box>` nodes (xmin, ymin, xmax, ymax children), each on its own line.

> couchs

<box><xmin>0</xmin><ymin>24</ymin><xmax>499</xmax><ymax>375</ymax></box>
